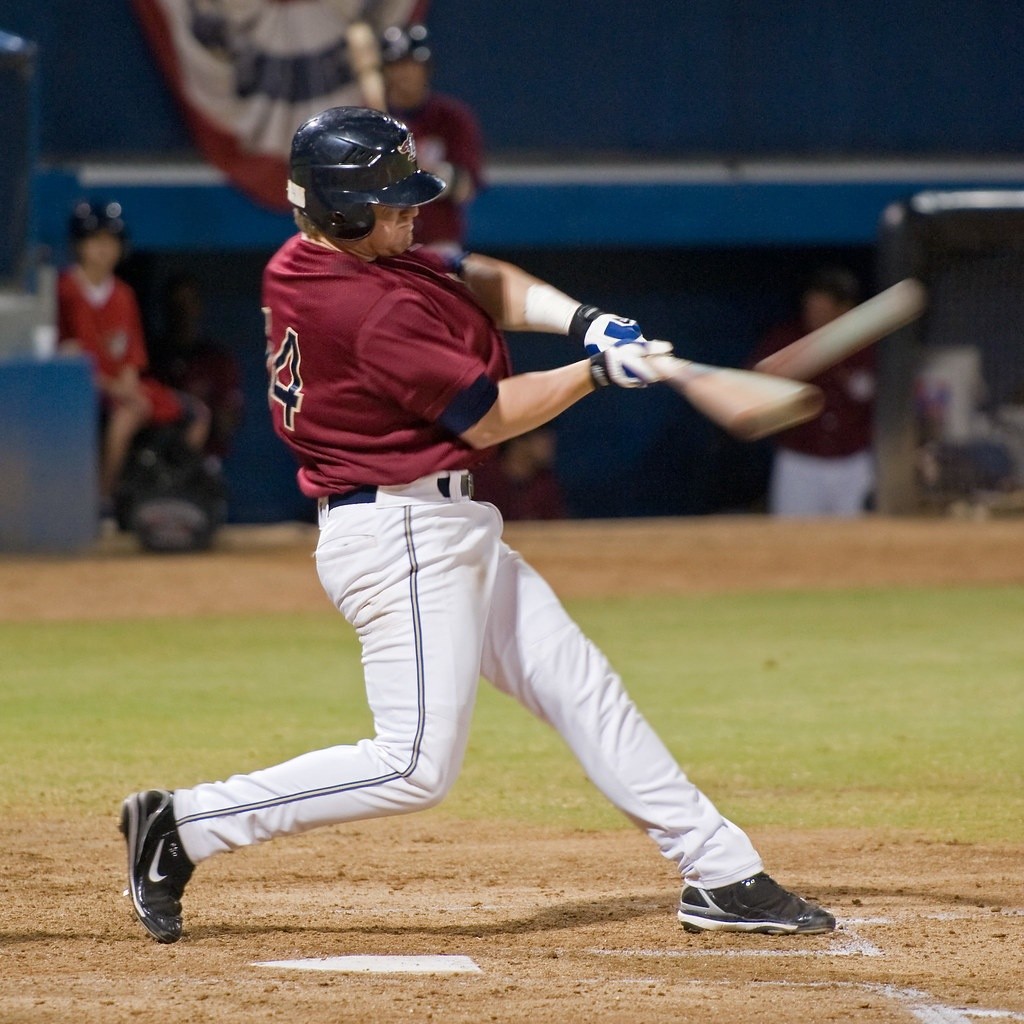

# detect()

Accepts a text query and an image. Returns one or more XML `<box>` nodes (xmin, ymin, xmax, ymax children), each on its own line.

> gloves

<box><xmin>567</xmin><ymin>304</ymin><xmax>647</xmax><ymax>356</ymax></box>
<box><xmin>591</xmin><ymin>341</ymin><xmax>673</xmax><ymax>389</ymax></box>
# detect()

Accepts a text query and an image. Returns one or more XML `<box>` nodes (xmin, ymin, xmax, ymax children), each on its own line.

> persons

<box><xmin>54</xmin><ymin>199</ymin><xmax>205</xmax><ymax>527</ymax></box>
<box><xmin>379</xmin><ymin>24</ymin><xmax>482</xmax><ymax>256</ymax></box>
<box><xmin>470</xmin><ymin>426</ymin><xmax>574</xmax><ymax>522</ymax></box>
<box><xmin>116</xmin><ymin>107</ymin><xmax>835</xmax><ymax>934</ymax></box>
<box><xmin>144</xmin><ymin>284</ymin><xmax>244</xmax><ymax>524</ymax></box>
<box><xmin>759</xmin><ymin>288</ymin><xmax>882</xmax><ymax>517</ymax></box>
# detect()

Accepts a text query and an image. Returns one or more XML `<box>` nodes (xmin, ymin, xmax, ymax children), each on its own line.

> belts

<box><xmin>328</xmin><ymin>473</ymin><xmax>474</xmax><ymax>511</ymax></box>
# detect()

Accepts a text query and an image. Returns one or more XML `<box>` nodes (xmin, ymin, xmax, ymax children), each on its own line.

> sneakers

<box><xmin>120</xmin><ymin>789</ymin><xmax>196</xmax><ymax>942</ymax></box>
<box><xmin>677</xmin><ymin>872</ymin><xmax>835</xmax><ymax>936</ymax></box>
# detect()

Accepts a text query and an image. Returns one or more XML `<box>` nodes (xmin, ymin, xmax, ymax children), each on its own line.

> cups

<box><xmin>30</xmin><ymin>325</ymin><xmax>56</xmax><ymax>356</ymax></box>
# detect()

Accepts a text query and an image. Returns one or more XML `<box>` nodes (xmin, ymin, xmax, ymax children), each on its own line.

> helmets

<box><xmin>381</xmin><ymin>25</ymin><xmax>435</xmax><ymax>66</ymax></box>
<box><xmin>287</xmin><ymin>105</ymin><xmax>446</xmax><ymax>241</ymax></box>
<box><xmin>72</xmin><ymin>201</ymin><xmax>126</xmax><ymax>238</ymax></box>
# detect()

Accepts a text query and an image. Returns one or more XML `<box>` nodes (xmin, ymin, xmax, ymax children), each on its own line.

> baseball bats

<box><xmin>341</xmin><ymin>18</ymin><xmax>386</xmax><ymax>118</ymax></box>
<box><xmin>649</xmin><ymin>349</ymin><xmax>822</xmax><ymax>442</ymax></box>
